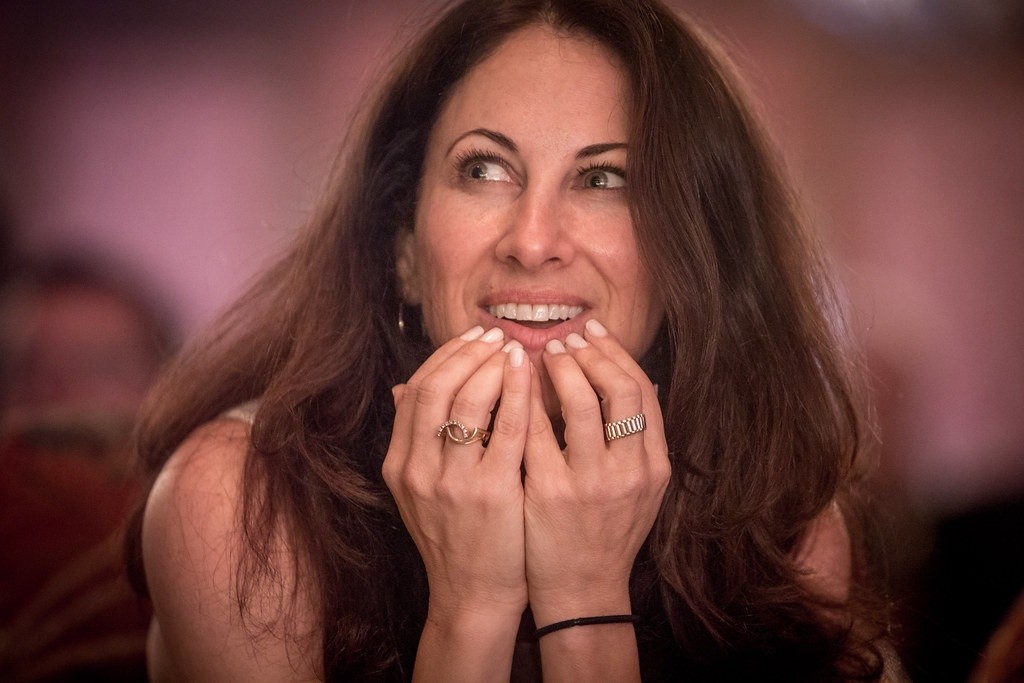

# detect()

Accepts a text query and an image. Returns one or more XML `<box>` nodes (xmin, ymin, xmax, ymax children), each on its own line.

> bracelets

<box><xmin>534</xmin><ymin>614</ymin><xmax>637</xmax><ymax>642</ymax></box>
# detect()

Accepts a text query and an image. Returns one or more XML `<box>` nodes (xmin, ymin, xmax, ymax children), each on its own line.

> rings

<box><xmin>437</xmin><ymin>420</ymin><xmax>490</xmax><ymax>447</ymax></box>
<box><xmin>604</xmin><ymin>414</ymin><xmax>646</xmax><ymax>441</ymax></box>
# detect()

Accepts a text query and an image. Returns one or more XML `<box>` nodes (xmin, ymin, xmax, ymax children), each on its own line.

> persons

<box><xmin>0</xmin><ymin>254</ymin><xmax>176</xmax><ymax>682</ymax></box>
<box><xmin>117</xmin><ymin>0</ymin><xmax>910</xmax><ymax>682</ymax></box>
